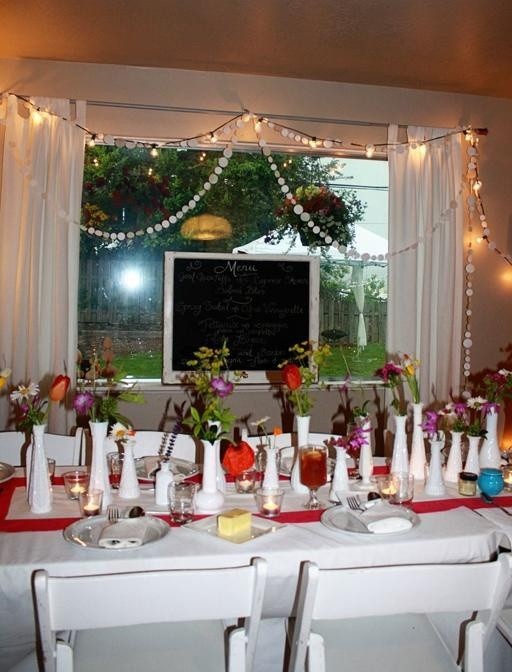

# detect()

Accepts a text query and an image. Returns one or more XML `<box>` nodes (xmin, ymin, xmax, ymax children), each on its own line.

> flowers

<box><xmin>271</xmin><ymin>182</ymin><xmax>346</xmax><ymax>245</ymax></box>
<box><xmin>80</xmin><ymin>145</ymin><xmax>183</xmax><ymax>232</ymax></box>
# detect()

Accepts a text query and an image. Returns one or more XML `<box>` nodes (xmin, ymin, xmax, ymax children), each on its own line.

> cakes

<box><xmin>217</xmin><ymin>508</ymin><xmax>251</xmax><ymax>537</ymax></box>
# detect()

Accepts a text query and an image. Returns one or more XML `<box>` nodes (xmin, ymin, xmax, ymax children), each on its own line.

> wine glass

<box><xmin>301</xmin><ymin>444</ymin><xmax>328</xmax><ymax>509</ymax></box>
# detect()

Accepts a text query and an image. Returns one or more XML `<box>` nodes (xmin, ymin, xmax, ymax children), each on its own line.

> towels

<box><xmin>98</xmin><ymin>518</ymin><xmax>150</xmax><ymax>549</ymax></box>
<box><xmin>358</xmin><ymin>504</ymin><xmax>414</xmax><ymax>534</ymax></box>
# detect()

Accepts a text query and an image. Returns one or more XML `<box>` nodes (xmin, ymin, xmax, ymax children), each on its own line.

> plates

<box><xmin>320</xmin><ymin>502</ymin><xmax>421</xmax><ymax>535</ymax></box>
<box><xmin>61</xmin><ymin>512</ymin><xmax>171</xmax><ymax>551</ymax></box>
<box><xmin>0</xmin><ymin>461</ymin><xmax>16</xmax><ymax>485</ymax></box>
<box><xmin>134</xmin><ymin>455</ymin><xmax>200</xmax><ymax>481</ymax></box>
<box><xmin>277</xmin><ymin>445</ymin><xmax>334</xmax><ymax>477</ymax></box>
<box><xmin>180</xmin><ymin>509</ymin><xmax>287</xmax><ymax>544</ymax></box>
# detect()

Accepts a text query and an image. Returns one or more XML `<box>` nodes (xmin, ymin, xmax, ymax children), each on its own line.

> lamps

<box><xmin>180</xmin><ymin>213</ymin><xmax>233</xmax><ymax>252</ymax></box>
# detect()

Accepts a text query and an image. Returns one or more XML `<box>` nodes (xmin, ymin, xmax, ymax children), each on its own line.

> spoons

<box><xmin>128</xmin><ymin>506</ymin><xmax>145</xmax><ymax>543</ymax></box>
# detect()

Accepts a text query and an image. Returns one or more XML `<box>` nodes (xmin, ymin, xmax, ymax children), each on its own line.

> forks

<box><xmin>108</xmin><ymin>507</ymin><xmax>119</xmax><ymax>544</ymax></box>
<box><xmin>346</xmin><ymin>496</ymin><xmax>365</xmax><ymax>512</ymax></box>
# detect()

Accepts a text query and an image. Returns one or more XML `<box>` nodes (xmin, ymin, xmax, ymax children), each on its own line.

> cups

<box><xmin>167</xmin><ymin>481</ymin><xmax>195</xmax><ymax>521</ymax></box>
<box><xmin>110</xmin><ymin>453</ymin><xmax>124</xmax><ymax>489</ymax></box>
<box><xmin>47</xmin><ymin>457</ymin><xmax>56</xmax><ymax>483</ymax></box>
<box><xmin>502</xmin><ymin>464</ymin><xmax>512</xmax><ymax>492</ymax></box>
<box><xmin>253</xmin><ymin>486</ymin><xmax>284</xmax><ymax>518</ymax></box>
<box><xmin>62</xmin><ymin>469</ymin><xmax>91</xmax><ymax>500</ymax></box>
<box><xmin>77</xmin><ymin>487</ymin><xmax>104</xmax><ymax>516</ymax></box>
<box><xmin>374</xmin><ymin>473</ymin><xmax>401</xmax><ymax>506</ymax></box>
<box><xmin>393</xmin><ymin>470</ymin><xmax>415</xmax><ymax>503</ymax></box>
<box><xmin>234</xmin><ymin>467</ymin><xmax>257</xmax><ymax>494</ymax></box>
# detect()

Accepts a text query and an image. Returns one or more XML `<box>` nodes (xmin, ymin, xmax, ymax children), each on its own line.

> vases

<box><xmin>118</xmin><ymin>207</ymin><xmax>137</xmax><ymax>225</ymax></box>
<box><xmin>298</xmin><ymin>229</ymin><xmax>332</xmax><ymax>246</ymax></box>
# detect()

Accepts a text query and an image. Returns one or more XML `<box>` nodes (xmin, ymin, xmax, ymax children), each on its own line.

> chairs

<box><xmin>33</xmin><ymin>557</ymin><xmax>268</xmax><ymax>672</ymax></box>
<box><xmin>284</xmin><ymin>551</ymin><xmax>512</xmax><ymax>672</ymax></box>
